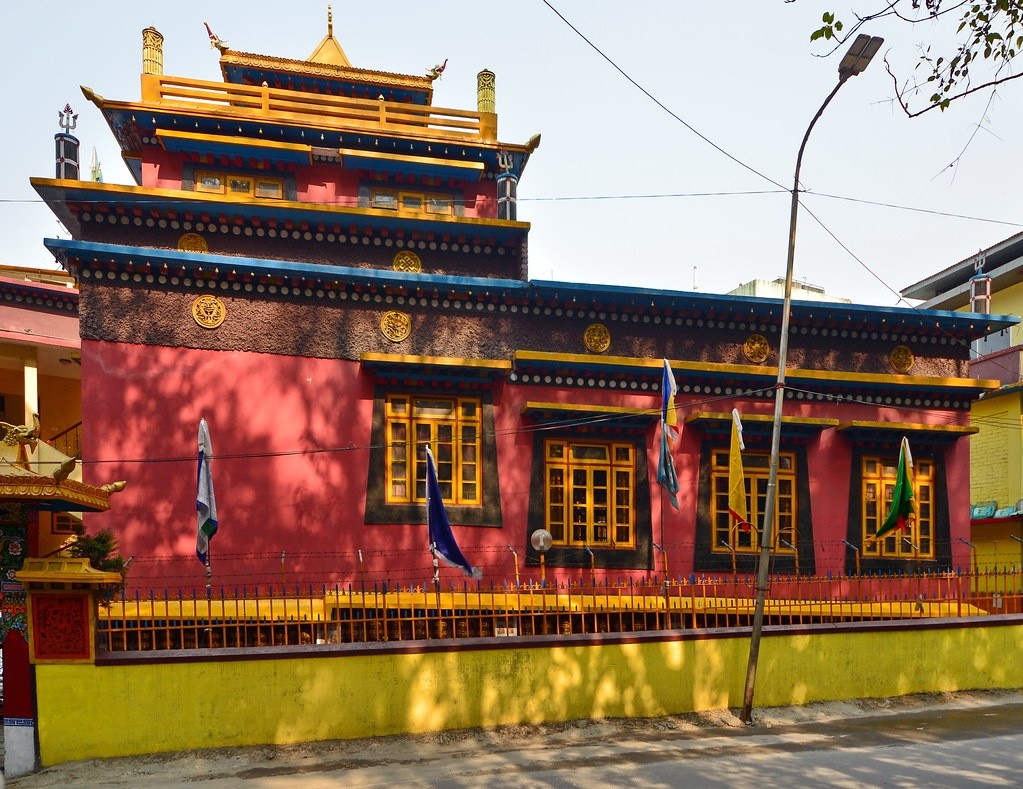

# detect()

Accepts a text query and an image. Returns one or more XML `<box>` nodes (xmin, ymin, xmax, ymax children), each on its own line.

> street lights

<box><xmin>739</xmin><ymin>33</ymin><xmax>885</xmax><ymax>725</ymax></box>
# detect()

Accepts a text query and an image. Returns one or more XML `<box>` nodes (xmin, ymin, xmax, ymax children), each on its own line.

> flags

<box><xmin>728</xmin><ymin>408</ymin><xmax>750</xmax><ymax>533</ymax></box>
<box><xmin>195</xmin><ymin>419</ymin><xmax>219</xmax><ymax>564</ymax></box>
<box><xmin>426</xmin><ymin>449</ymin><xmax>481</xmax><ymax>577</ymax></box>
<box><xmin>656</xmin><ymin>358</ymin><xmax>679</xmax><ymax>510</ymax></box>
<box><xmin>864</xmin><ymin>437</ymin><xmax>915</xmax><ymax>548</ymax></box>
